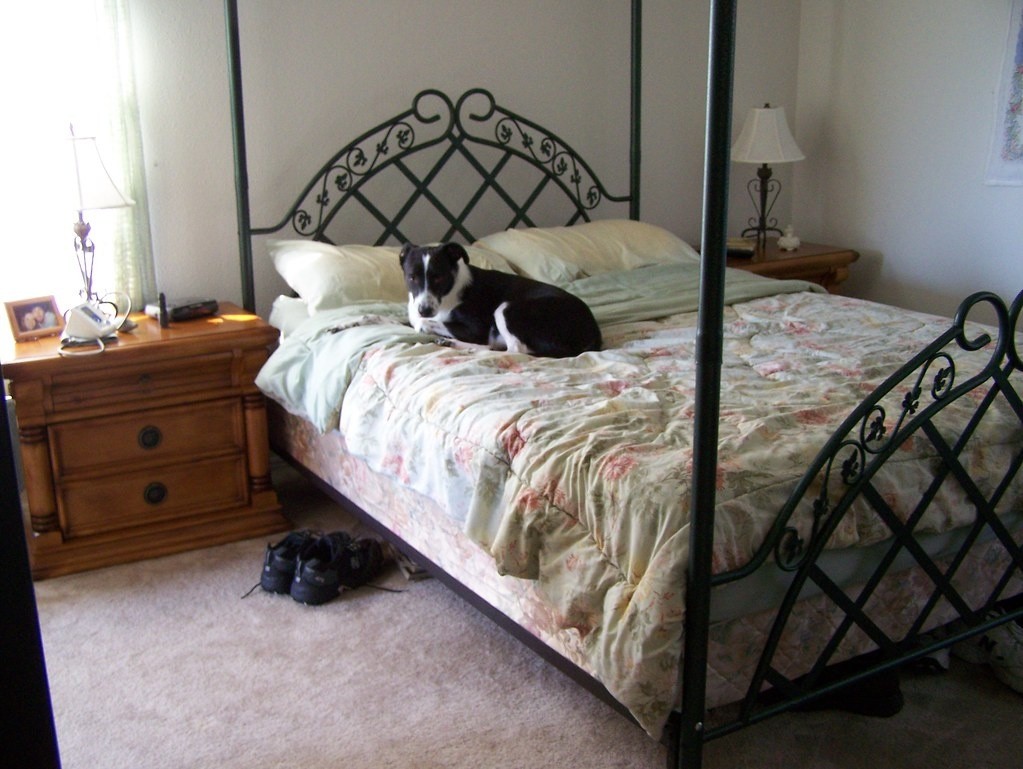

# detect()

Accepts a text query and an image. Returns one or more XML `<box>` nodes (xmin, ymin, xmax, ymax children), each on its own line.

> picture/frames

<box><xmin>4</xmin><ymin>294</ymin><xmax>67</xmax><ymax>343</ymax></box>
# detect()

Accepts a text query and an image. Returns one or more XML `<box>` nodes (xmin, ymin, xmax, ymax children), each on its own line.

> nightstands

<box><xmin>0</xmin><ymin>299</ymin><xmax>289</xmax><ymax>581</ymax></box>
<box><xmin>692</xmin><ymin>239</ymin><xmax>861</xmax><ymax>299</ymax></box>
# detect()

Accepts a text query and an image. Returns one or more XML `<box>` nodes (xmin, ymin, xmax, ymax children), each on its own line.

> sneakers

<box><xmin>291</xmin><ymin>539</ymin><xmax>402</xmax><ymax>604</ymax></box>
<box><xmin>239</xmin><ymin>528</ymin><xmax>352</xmax><ymax>599</ymax></box>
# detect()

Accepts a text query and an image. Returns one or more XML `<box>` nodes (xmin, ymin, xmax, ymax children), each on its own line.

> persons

<box><xmin>21</xmin><ymin>311</ymin><xmax>40</xmax><ymax>332</ymax></box>
<box><xmin>32</xmin><ymin>305</ymin><xmax>57</xmax><ymax>328</ymax></box>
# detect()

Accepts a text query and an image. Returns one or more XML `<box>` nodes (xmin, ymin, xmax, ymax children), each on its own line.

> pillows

<box><xmin>471</xmin><ymin>218</ymin><xmax>700</xmax><ymax>289</ymax></box>
<box><xmin>263</xmin><ymin>238</ymin><xmax>517</xmax><ymax>321</ymax></box>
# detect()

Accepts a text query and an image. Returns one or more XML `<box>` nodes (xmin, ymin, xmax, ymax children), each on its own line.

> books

<box><xmin>729</xmin><ymin>235</ymin><xmax>756</xmax><ymax>250</ymax></box>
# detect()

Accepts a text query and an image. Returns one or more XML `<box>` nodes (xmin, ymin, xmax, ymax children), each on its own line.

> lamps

<box><xmin>731</xmin><ymin>101</ymin><xmax>806</xmax><ymax>254</ymax></box>
<box><xmin>43</xmin><ymin>123</ymin><xmax>136</xmax><ymax>342</ymax></box>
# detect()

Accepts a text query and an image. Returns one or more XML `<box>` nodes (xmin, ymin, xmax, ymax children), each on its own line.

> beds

<box><xmin>224</xmin><ymin>0</ymin><xmax>1023</xmax><ymax>769</ymax></box>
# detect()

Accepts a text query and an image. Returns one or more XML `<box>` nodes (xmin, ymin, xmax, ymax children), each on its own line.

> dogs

<box><xmin>398</xmin><ymin>243</ymin><xmax>603</xmax><ymax>359</ymax></box>
